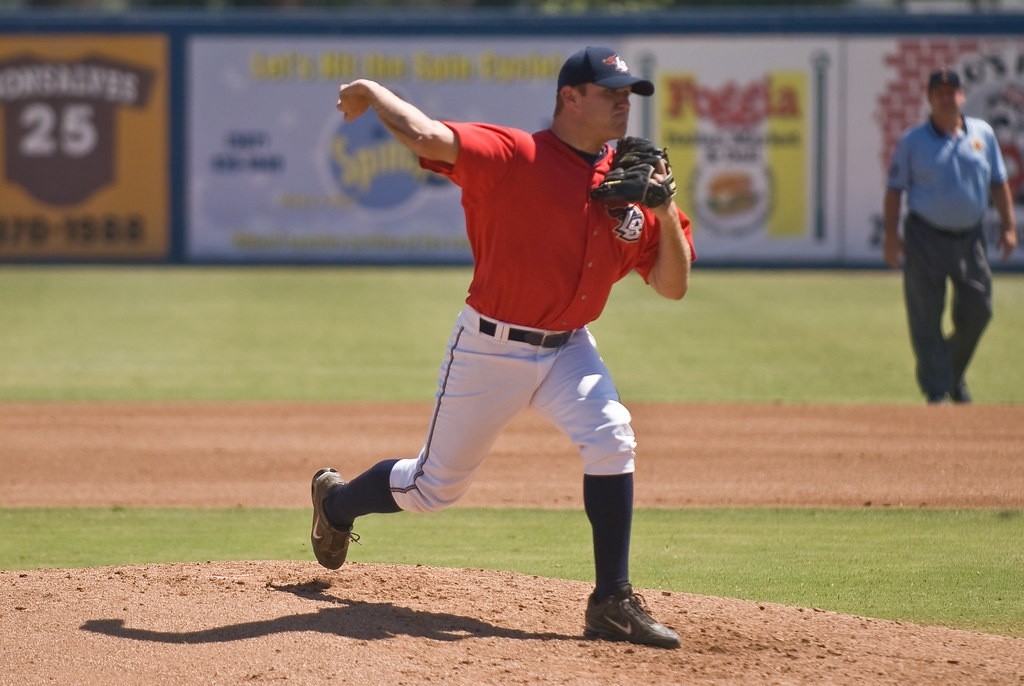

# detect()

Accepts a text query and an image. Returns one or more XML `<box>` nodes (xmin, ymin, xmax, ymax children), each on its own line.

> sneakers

<box><xmin>311</xmin><ymin>468</ymin><xmax>361</xmax><ymax>569</ymax></box>
<box><xmin>583</xmin><ymin>583</ymin><xmax>681</xmax><ymax>648</ymax></box>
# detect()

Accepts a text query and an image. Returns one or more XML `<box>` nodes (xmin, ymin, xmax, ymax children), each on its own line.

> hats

<box><xmin>930</xmin><ymin>68</ymin><xmax>959</xmax><ymax>87</ymax></box>
<box><xmin>558</xmin><ymin>46</ymin><xmax>654</xmax><ymax>96</ymax></box>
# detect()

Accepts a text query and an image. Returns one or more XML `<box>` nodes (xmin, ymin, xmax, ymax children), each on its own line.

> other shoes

<box><xmin>928</xmin><ymin>396</ymin><xmax>945</xmax><ymax>404</ymax></box>
<box><xmin>946</xmin><ymin>380</ymin><xmax>970</xmax><ymax>401</ymax></box>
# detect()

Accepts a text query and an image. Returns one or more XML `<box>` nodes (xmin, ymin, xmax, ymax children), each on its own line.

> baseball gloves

<box><xmin>589</xmin><ymin>137</ymin><xmax>678</xmax><ymax>207</ymax></box>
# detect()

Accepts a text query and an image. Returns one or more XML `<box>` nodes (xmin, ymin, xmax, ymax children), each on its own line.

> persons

<box><xmin>882</xmin><ymin>67</ymin><xmax>1018</xmax><ymax>405</ymax></box>
<box><xmin>310</xmin><ymin>42</ymin><xmax>693</xmax><ymax>649</ymax></box>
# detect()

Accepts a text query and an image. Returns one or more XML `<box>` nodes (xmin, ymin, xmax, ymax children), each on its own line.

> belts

<box><xmin>480</xmin><ymin>318</ymin><xmax>572</xmax><ymax>347</ymax></box>
